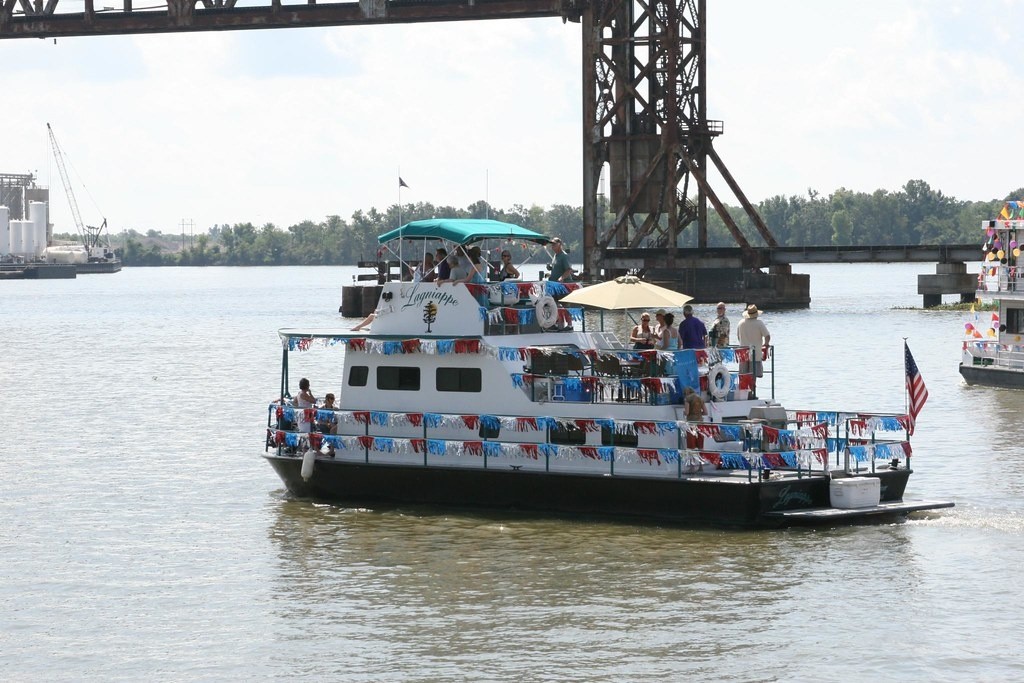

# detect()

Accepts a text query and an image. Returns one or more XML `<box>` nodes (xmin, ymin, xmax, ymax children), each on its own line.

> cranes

<box><xmin>45</xmin><ymin>121</ymin><xmax>110</xmax><ymax>261</ymax></box>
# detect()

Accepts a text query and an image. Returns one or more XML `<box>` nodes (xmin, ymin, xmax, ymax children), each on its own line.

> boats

<box><xmin>259</xmin><ymin>217</ymin><xmax>958</xmax><ymax>527</ymax></box>
<box><xmin>956</xmin><ymin>200</ymin><xmax>1024</xmax><ymax>389</ymax></box>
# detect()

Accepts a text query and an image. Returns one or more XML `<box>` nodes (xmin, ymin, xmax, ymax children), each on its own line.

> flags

<box><xmin>399</xmin><ymin>178</ymin><xmax>407</xmax><ymax>186</ymax></box>
<box><xmin>904</xmin><ymin>341</ymin><xmax>928</xmax><ymax>435</ymax></box>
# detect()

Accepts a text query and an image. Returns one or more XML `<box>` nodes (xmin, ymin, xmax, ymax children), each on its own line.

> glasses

<box><xmin>641</xmin><ymin>319</ymin><xmax>651</xmax><ymax>322</ymax></box>
<box><xmin>502</xmin><ymin>255</ymin><xmax>510</xmax><ymax>258</ymax></box>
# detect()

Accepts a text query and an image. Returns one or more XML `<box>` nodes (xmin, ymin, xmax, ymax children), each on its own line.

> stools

<box><xmin>610</xmin><ymin>362</ymin><xmax>642</xmax><ymax>403</ymax></box>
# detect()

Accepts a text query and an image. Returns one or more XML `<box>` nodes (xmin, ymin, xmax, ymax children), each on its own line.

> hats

<box><xmin>743</xmin><ymin>304</ymin><xmax>763</xmax><ymax>319</ymax></box>
<box><xmin>549</xmin><ymin>237</ymin><xmax>562</xmax><ymax>244</ymax></box>
<box><xmin>654</xmin><ymin>309</ymin><xmax>667</xmax><ymax>316</ymax></box>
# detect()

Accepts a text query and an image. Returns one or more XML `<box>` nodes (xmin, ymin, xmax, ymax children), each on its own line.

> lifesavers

<box><xmin>40</xmin><ymin>254</ymin><xmax>47</xmax><ymax>261</ymax></box>
<box><xmin>535</xmin><ymin>296</ymin><xmax>558</xmax><ymax>329</ymax></box>
<box><xmin>708</xmin><ymin>364</ymin><xmax>730</xmax><ymax>397</ymax></box>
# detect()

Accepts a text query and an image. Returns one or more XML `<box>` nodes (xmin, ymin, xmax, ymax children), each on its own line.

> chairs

<box><xmin>487</xmin><ymin>281</ymin><xmax>521</xmax><ymax>336</ymax></box>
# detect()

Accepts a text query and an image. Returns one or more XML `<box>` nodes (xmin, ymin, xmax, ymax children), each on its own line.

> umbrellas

<box><xmin>558</xmin><ymin>275</ymin><xmax>694</xmax><ymax>349</ymax></box>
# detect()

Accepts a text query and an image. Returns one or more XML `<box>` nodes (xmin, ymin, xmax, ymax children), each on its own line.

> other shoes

<box><xmin>546</xmin><ymin>324</ymin><xmax>560</xmax><ymax>332</ymax></box>
<box><xmin>560</xmin><ymin>325</ymin><xmax>574</xmax><ymax>332</ymax></box>
<box><xmin>748</xmin><ymin>394</ymin><xmax>759</xmax><ymax>400</ymax></box>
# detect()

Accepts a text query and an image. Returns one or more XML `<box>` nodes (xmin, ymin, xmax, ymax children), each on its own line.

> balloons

<box><xmin>965</xmin><ymin>323</ymin><xmax>973</xmax><ymax>335</ymax></box>
<box><xmin>1015</xmin><ymin>336</ymin><xmax>1020</xmax><ymax>341</ymax></box>
<box><xmin>986</xmin><ymin>229</ymin><xmax>1024</xmax><ymax>264</ymax></box>
<box><xmin>987</xmin><ymin>329</ymin><xmax>994</xmax><ymax>336</ymax></box>
<box><xmin>994</xmin><ymin>322</ymin><xmax>999</xmax><ymax>328</ymax></box>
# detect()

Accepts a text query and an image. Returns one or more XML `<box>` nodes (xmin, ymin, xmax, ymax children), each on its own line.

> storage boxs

<box><xmin>668</xmin><ymin>375</ymin><xmax>710</xmax><ymax>405</ymax></box>
<box><xmin>552</xmin><ymin>380</ymin><xmax>594</xmax><ymax>402</ymax></box>
<box><xmin>830</xmin><ymin>477</ymin><xmax>880</xmax><ymax>509</ymax></box>
<box><xmin>727</xmin><ymin>389</ymin><xmax>752</xmax><ymax>401</ymax></box>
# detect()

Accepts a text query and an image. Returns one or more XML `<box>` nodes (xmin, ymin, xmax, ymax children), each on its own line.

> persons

<box><xmin>629</xmin><ymin>309</ymin><xmax>683</xmax><ymax>350</ymax></box>
<box><xmin>709</xmin><ymin>302</ymin><xmax>730</xmax><ymax>347</ymax></box>
<box><xmin>292</xmin><ymin>378</ymin><xmax>317</xmax><ymax>433</ymax></box>
<box><xmin>350</xmin><ymin>313</ymin><xmax>374</xmax><ymax>331</ymax></box>
<box><xmin>547</xmin><ymin>238</ymin><xmax>571</xmax><ymax>281</ymax></box>
<box><xmin>409</xmin><ymin>244</ymin><xmax>486</xmax><ymax>286</ymax></box>
<box><xmin>317</xmin><ymin>393</ymin><xmax>338</xmax><ymax>433</ymax></box>
<box><xmin>494</xmin><ymin>250</ymin><xmax>520</xmax><ymax>280</ymax></box>
<box><xmin>679</xmin><ymin>305</ymin><xmax>707</xmax><ymax>349</ymax></box>
<box><xmin>737</xmin><ymin>305</ymin><xmax>770</xmax><ymax>377</ymax></box>
<box><xmin>683</xmin><ymin>387</ymin><xmax>708</xmax><ymax>475</ymax></box>
